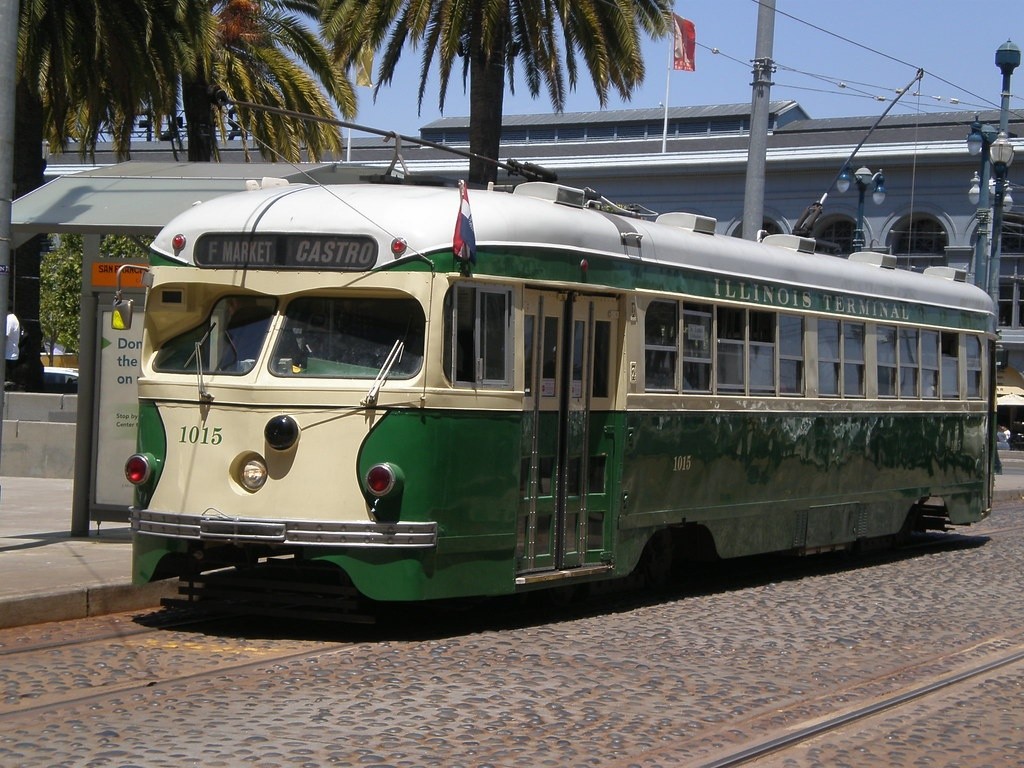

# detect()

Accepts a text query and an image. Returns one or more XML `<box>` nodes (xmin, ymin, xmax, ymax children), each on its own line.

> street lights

<box><xmin>965</xmin><ymin>116</ymin><xmax>998</xmax><ymax>293</ymax></box>
<box><xmin>989</xmin><ymin>129</ymin><xmax>1016</xmax><ymax>314</ymax></box>
<box><xmin>995</xmin><ymin>38</ymin><xmax>1022</xmax><ymax>133</ymax></box>
<box><xmin>835</xmin><ymin>164</ymin><xmax>885</xmax><ymax>255</ymax></box>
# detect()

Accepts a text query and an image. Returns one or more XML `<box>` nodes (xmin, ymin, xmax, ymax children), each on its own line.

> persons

<box><xmin>224</xmin><ymin>305</ymin><xmax>301</xmax><ymax>371</ymax></box>
<box><xmin>997</xmin><ymin>426</ymin><xmax>1010</xmax><ymax>442</ymax></box>
<box><xmin>5</xmin><ymin>301</ymin><xmax>20</xmax><ymax>368</ymax></box>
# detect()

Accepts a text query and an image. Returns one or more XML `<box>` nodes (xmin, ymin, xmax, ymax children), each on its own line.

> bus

<box><xmin>112</xmin><ymin>70</ymin><xmax>1003</xmax><ymax>623</ymax></box>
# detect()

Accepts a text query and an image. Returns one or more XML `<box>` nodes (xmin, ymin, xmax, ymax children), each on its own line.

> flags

<box><xmin>453</xmin><ymin>183</ymin><xmax>479</xmax><ymax>266</ymax></box>
<box><xmin>674</xmin><ymin>15</ymin><xmax>696</xmax><ymax>71</ymax></box>
